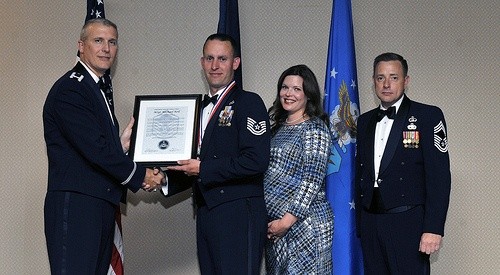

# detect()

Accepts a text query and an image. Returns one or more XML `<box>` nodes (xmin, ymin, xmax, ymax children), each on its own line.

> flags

<box><xmin>323</xmin><ymin>0</ymin><xmax>364</xmax><ymax>275</ymax></box>
<box><xmin>75</xmin><ymin>0</ymin><xmax>125</xmax><ymax>275</ymax></box>
<box><xmin>217</xmin><ymin>0</ymin><xmax>242</xmax><ymax>90</ymax></box>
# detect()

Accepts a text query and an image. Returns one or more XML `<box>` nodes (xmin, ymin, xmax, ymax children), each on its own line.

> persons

<box><xmin>141</xmin><ymin>33</ymin><xmax>271</xmax><ymax>275</ymax></box>
<box><xmin>356</xmin><ymin>53</ymin><xmax>451</xmax><ymax>275</ymax></box>
<box><xmin>263</xmin><ymin>65</ymin><xmax>334</xmax><ymax>275</ymax></box>
<box><xmin>42</xmin><ymin>18</ymin><xmax>167</xmax><ymax>275</ymax></box>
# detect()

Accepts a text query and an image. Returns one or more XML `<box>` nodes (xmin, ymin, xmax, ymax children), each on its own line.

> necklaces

<box><xmin>285</xmin><ymin>113</ymin><xmax>308</xmax><ymax>123</ymax></box>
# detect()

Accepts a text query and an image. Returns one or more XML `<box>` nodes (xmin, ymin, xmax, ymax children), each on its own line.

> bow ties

<box><xmin>376</xmin><ymin>106</ymin><xmax>396</xmax><ymax>123</ymax></box>
<box><xmin>96</xmin><ymin>78</ymin><xmax>108</xmax><ymax>93</ymax></box>
<box><xmin>201</xmin><ymin>94</ymin><xmax>219</xmax><ymax>107</ymax></box>
